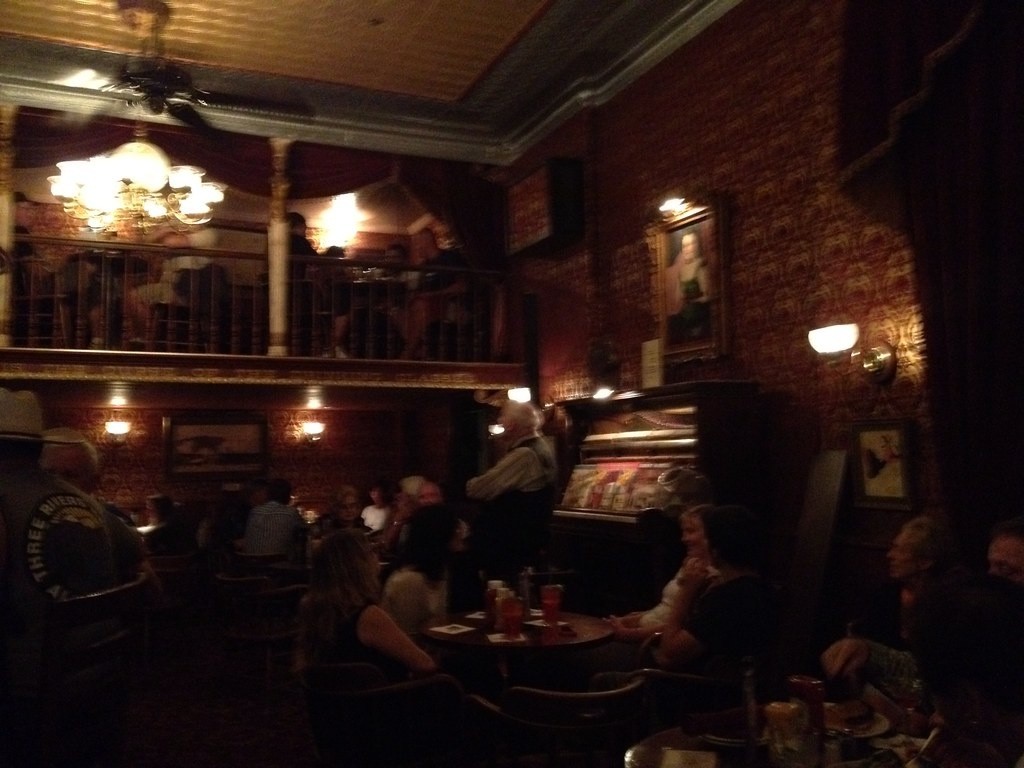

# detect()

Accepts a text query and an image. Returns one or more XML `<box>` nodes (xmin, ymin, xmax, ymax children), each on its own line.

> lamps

<box><xmin>806</xmin><ymin>322</ymin><xmax>899</xmax><ymax>383</ymax></box>
<box><xmin>591</xmin><ymin>383</ymin><xmax>618</xmax><ymax>401</ymax></box>
<box><xmin>44</xmin><ymin>129</ymin><xmax>227</xmax><ymax>241</ymax></box>
<box><xmin>848</xmin><ymin>415</ymin><xmax>920</xmax><ymax>514</ymax></box>
<box><xmin>104</xmin><ymin>421</ymin><xmax>132</xmax><ymax>446</ymax></box>
<box><xmin>298</xmin><ymin>420</ymin><xmax>328</xmax><ymax>444</ymax></box>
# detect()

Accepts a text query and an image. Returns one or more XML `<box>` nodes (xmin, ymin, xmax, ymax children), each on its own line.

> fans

<box><xmin>44</xmin><ymin>8</ymin><xmax>315</xmax><ymax>169</ymax></box>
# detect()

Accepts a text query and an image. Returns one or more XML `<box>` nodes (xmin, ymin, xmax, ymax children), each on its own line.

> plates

<box><xmin>812</xmin><ymin>702</ymin><xmax>889</xmax><ymax>740</ymax></box>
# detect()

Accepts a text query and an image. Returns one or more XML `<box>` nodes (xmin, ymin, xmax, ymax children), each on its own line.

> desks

<box><xmin>619</xmin><ymin>696</ymin><xmax>1013</xmax><ymax>768</ymax></box>
<box><xmin>330</xmin><ymin>276</ymin><xmax>410</xmax><ymax>358</ymax></box>
<box><xmin>68</xmin><ymin>248</ymin><xmax>148</xmax><ymax>348</ymax></box>
<box><xmin>418</xmin><ymin>605</ymin><xmax>617</xmax><ymax>683</ymax></box>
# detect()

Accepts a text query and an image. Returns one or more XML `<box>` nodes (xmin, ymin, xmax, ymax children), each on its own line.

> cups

<box><xmin>486</xmin><ymin>578</ymin><xmax>563</xmax><ymax>639</ymax></box>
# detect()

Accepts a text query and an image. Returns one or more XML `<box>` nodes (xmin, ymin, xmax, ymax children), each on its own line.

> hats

<box><xmin>0</xmin><ymin>385</ymin><xmax>90</xmax><ymax>445</ymax></box>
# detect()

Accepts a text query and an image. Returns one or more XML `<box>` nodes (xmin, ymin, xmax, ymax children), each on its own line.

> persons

<box><xmin>0</xmin><ymin>388</ymin><xmax>131</xmax><ymax>768</ymax></box>
<box><xmin>668</xmin><ymin>226</ymin><xmax>712</xmax><ymax>344</ymax></box>
<box><xmin>10</xmin><ymin>191</ymin><xmax>490</xmax><ymax>363</ymax></box>
<box><xmin>148</xmin><ymin>401</ymin><xmax>1024</xmax><ymax>768</ymax></box>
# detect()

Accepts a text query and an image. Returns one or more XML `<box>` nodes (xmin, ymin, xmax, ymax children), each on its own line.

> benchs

<box><xmin>427</xmin><ymin>276</ymin><xmax>482</xmax><ymax>357</ymax></box>
<box><xmin>259</xmin><ymin>276</ymin><xmax>336</xmax><ymax>356</ymax></box>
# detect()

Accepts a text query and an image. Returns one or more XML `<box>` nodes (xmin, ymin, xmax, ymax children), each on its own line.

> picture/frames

<box><xmin>646</xmin><ymin>190</ymin><xmax>730</xmax><ymax>368</ymax></box>
<box><xmin>160</xmin><ymin>409</ymin><xmax>271</xmax><ymax>467</ymax></box>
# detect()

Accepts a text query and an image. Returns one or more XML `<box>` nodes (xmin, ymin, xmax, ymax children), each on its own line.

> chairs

<box><xmin>482</xmin><ymin>669</ymin><xmax>647</xmax><ymax>768</ymax></box>
<box><xmin>299</xmin><ymin>652</ymin><xmax>490</xmax><ymax>768</ymax></box>
<box><xmin>632</xmin><ymin>666</ymin><xmax>766</xmax><ymax>707</ymax></box>
<box><xmin>9</xmin><ymin>569</ymin><xmax>151</xmax><ymax>768</ymax></box>
<box><xmin>13</xmin><ymin>250</ymin><xmax>72</xmax><ymax>348</ymax></box>
<box><xmin>149</xmin><ymin>263</ymin><xmax>227</xmax><ymax>352</ymax></box>
<box><xmin>214</xmin><ymin>568</ymin><xmax>311</xmax><ymax>673</ymax></box>
